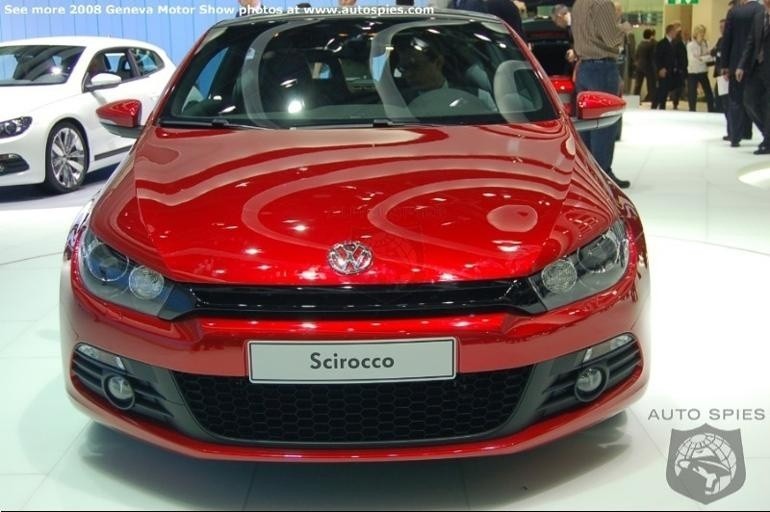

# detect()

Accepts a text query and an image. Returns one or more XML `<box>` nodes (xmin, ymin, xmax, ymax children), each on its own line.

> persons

<box><xmin>235</xmin><ymin>1</ymin><xmax>416</xmax><ymax>21</ymax></box>
<box><xmin>441</xmin><ymin>1</ymin><xmax>525</xmax><ymax>97</ymax></box>
<box><xmin>651</xmin><ymin>22</ymin><xmax>677</xmax><ymax>110</ymax></box>
<box><xmin>550</xmin><ymin>1</ymin><xmax>658</xmax><ymax>188</ymax></box>
<box><xmin>685</xmin><ymin>24</ymin><xmax>718</xmax><ymax>113</ymax></box>
<box><xmin>706</xmin><ymin>19</ymin><xmax>728</xmax><ymax>113</ymax></box>
<box><xmin>713</xmin><ymin>1</ymin><xmax>770</xmax><ymax>155</ymax></box>
<box><xmin>670</xmin><ymin>23</ymin><xmax>688</xmax><ymax>110</ymax></box>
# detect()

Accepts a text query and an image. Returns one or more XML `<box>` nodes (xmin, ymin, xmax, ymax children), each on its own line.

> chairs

<box><xmin>308</xmin><ymin>49</ymin><xmax>353</xmax><ymax>107</ymax></box>
<box><xmin>117</xmin><ymin>54</ymin><xmax>143</xmax><ymax>81</ymax></box>
<box><xmin>377</xmin><ymin>50</ymin><xmax>411</xmax><ymax>95</ymax></box>
<box><xmin>231</xmin><ymin>52</ymin><xmax>312</xmax><ymax>114</ymax></box>
<box><xmin>13</xmin><ymin>57</ymin><xmax>49</xmax><ymax>81</ymax></box>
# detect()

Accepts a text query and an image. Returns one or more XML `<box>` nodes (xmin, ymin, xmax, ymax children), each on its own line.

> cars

<box><xmin>60</xmin><ymin>7</ymin><xmax>650</xmax><ymax>465</ymax></box>
<box><xmin>1</xmin><ymin>36</ymin><xmax>201</xmax><ymax>197</ymax></box>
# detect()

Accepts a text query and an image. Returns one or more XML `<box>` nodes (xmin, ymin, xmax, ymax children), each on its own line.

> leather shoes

<box><xmin>723</xmin><ymin>134</ymin><xmax>741</xmax><ymax>147</ymax></box>
<box><xmin>614</xmin><ymin>177</ymin><xmax>630</xmax><ymax>187</ymax></box>
<box><xmin>754</xmin><ymin>142</ymin><xmax>770</xmax><ymax>154</ymax></box>
